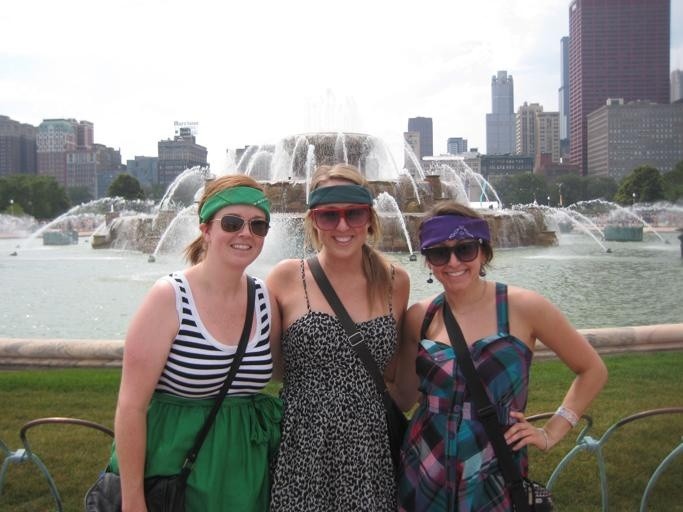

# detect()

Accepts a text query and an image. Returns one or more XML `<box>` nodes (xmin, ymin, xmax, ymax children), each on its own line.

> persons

<box><xmin>266</xmin><ymin>161</ymin><xmax>412</xmax><ymax>510</ymax></box>
<box><xmin>384</xmin><ymin>199</ymin><xmax>611</xmax><ymax>511</ymax></box>
<box><xmin>107</xmin><ymin>171</ymin><xmax>285</xmax><ymax>511</ymax></box>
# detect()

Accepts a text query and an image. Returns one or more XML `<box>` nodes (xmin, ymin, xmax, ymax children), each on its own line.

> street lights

<box><xmin>547</xmin><ymin>196</ymin><xmax>550</xmax><ymax>205</ymax></box>
<box><xmin>10</xmin><ymin>199</ymin><xmax>15</xmax><ymax>216</ymax></box>
<box><xmin>632</xmin><ymin>192</ymin><xmax>636</xmax><ymax>204</ymax></box>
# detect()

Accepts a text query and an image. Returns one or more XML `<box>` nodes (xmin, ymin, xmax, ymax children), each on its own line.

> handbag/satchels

<box><xmin>383</xmin><ymin>404</ymin><xmax>409</xmax><ymax>478</ymax></box>
<box><xmin>506</xmin><ymin>471</ymin><xmax>555</xmax><ymax>512</ymax></box>
<box><xmin>81</xmin><ymin>469</ymin><xmax>186</xmax><ymax>511</ymax></box>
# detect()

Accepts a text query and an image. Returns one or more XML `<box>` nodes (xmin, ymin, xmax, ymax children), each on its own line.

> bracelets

<box><xmin>555</xmin><ymin>405</ymin><xmax>581</xmax><ymax>429</ymax></box>
<box><xmin>538</xmin><ymin>426</ymin><xmax>549</xmax><ymax>453</ymax></box>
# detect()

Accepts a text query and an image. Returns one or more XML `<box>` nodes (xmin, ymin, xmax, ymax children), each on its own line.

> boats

<box><xmin>43</xmin><ymin>228</ymin><xmax>78</xmax><ymax>244</ymax></box>
<box><xmin>558</xmin><ymin>222</ymin><xmax>573</xmax><ymax>232</ymax></box>
<box><xmin>604</xmin><ymin>224</ymin><xmax>644</xmax><ymax>241</ymax></box>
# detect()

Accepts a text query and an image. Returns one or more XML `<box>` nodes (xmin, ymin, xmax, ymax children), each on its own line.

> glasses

<box><xmin>208</xmin><ymin>214</ymin><xmax>270</xmax><ymax>239</ymax></box>
<box><xmin>309</xmin><ymin>205</ymin><xmax>370</xmax><ymax>231</ymax></box>
<box><xmin>420</xmin><ymin>241</ymin><xmax>480</xmax><ymax>267</ymax></box>
